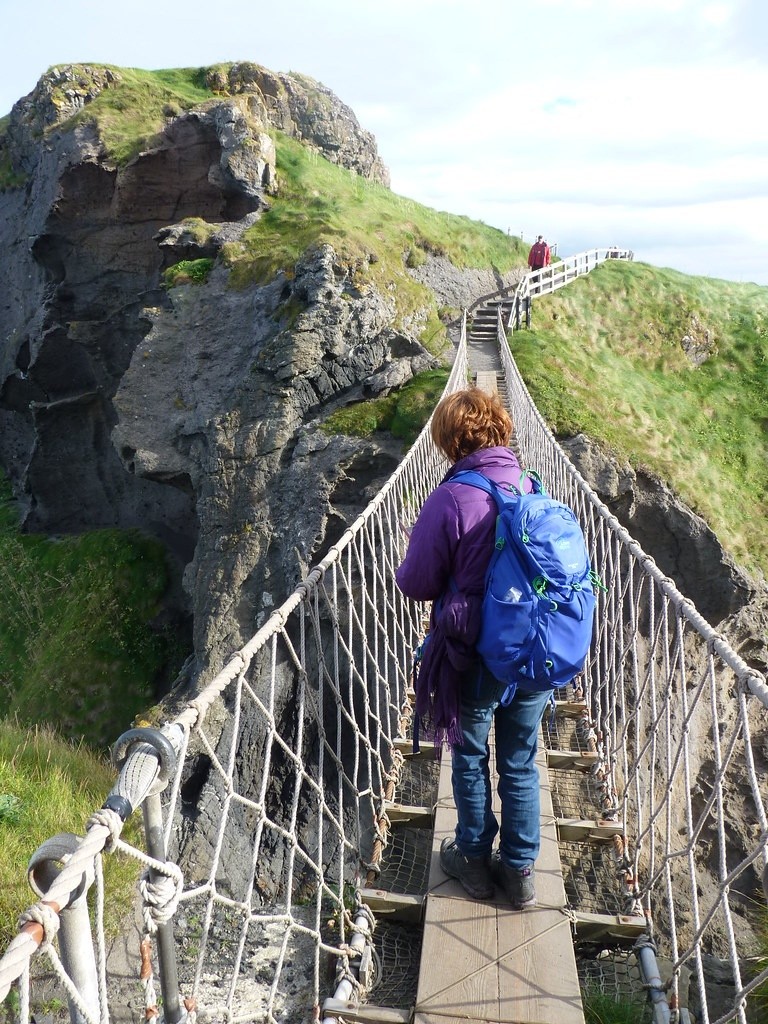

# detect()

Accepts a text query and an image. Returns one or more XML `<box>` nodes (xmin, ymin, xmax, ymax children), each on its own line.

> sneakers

<box><xmin>439</xmin><ymin>837</ymin><xmax>495</xmax><ymax>899</ymax></box>
<box><xmin>490</xmin><ymin>848</ymin><xmax>538</xmax><ymax>909</ymax></box>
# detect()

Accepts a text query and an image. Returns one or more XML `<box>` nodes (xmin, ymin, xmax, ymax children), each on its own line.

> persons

<box><xmin>393</xmin><ymin>390</ymin><xmax>554</xmax><ymax>909</ymax></box>
<box><xmin>605</xmin><ymin>246</ymin><xmax>633</xmax><ymax>260</ymax></box>
<box><xmin>527</xmin><ymin>235</ymin><xmax>551</xmax><ymax>294</ymax></box>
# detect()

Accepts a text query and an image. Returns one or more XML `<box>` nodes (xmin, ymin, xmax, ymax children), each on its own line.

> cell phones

<box><xmin>404</xmin><ymin>527</ymin><xmax>414</xmax><ymax>538</ymax></box>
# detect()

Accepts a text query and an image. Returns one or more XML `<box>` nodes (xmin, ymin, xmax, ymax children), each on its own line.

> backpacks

<box><xmin>449</xmin><ymin>468</ymin><xmax>596</xmax><ymax>691</ymax></box>
